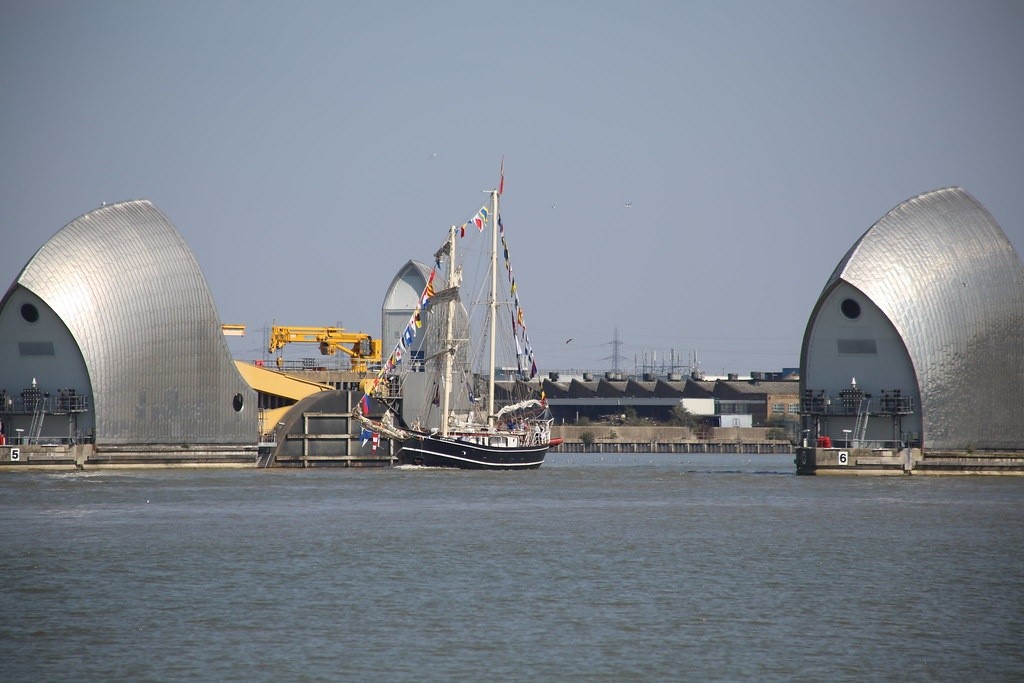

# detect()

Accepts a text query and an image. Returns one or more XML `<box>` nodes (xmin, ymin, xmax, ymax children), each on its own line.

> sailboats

<box><xmin>350</xmin><ymin>156</ymin><xmax>555</xmax><ymax>471</ymax></box>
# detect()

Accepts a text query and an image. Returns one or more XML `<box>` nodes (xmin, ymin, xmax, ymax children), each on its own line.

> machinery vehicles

<box><xmin>267</xmin><ymin>326</ymin><xmax>383</xmax><ymax>374</ymax></box>
